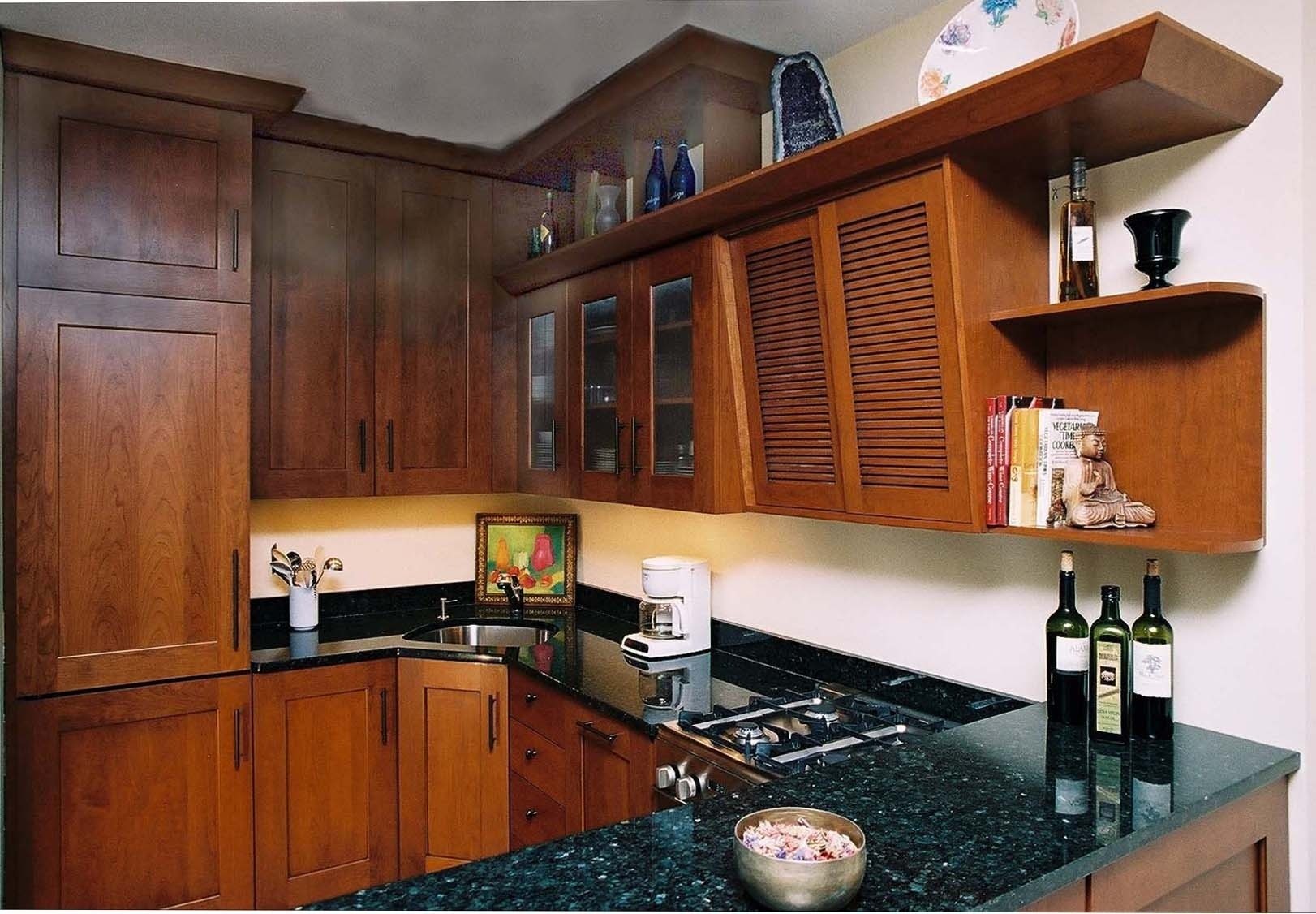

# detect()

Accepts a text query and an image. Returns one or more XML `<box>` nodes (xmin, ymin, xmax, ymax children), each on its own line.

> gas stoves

<box><xmin>652</xmin><ymin>682</ymin><xmax>948</xmax><ymax>809</ymax></box>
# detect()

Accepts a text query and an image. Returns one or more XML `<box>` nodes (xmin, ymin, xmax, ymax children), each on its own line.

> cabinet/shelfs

<box><xmin>0</xmin><ymin>24</ymin><xmax>1299</xmax><ymax>911</ymax></box>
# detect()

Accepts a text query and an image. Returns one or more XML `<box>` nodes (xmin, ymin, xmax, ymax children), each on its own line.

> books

<box><xmin>985</xmin><ymin>395</ymin><xmax>1099</xmax><ymax>530</ymax></box>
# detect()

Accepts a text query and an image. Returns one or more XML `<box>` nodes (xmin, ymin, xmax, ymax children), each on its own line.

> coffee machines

<box><xmin>620</xmin><ymin>554</ymin><xmax>711</xmax><ymax>659</ymax></box>
<box><xmin>619</xmin><ymin>651</ymin><xmax>713</xmax><ymax>725</ymax></box>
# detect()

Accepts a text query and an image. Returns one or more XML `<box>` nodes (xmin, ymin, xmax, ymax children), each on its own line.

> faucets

<box><xmin>496</xmin><ymin>572</ymin><xmax>524</xmax><ymax>617</ymax></box>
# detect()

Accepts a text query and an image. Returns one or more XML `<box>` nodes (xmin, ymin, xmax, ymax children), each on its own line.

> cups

<box><xmin>290</xmin><ymin>632</ymin><xmax>319</xmax><ymax>658</ymax></box>
<box><xmin>289</xmin><ymin>587</ymin><xmax>319</xmax><ymax>631</ymax></box>
<box><xmin>524</xmin><ymin>226</ymin><xmax>542</xmax><ymax>257</ymax></box>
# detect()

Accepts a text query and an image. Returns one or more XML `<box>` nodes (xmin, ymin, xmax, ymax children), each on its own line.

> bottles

<box><xmin>1045</xmin><ymin>550</ymin><xmax>1090</xmax><ymax>717</ymax></box>
<box><xmin>643</xmin><ymin>138</ymin><xmax>669</xmax><ymax>214</ymax></box>
<box><xmin>1088</xmin><ymin>584</ymin><xmax>1132</xmax><ymax>748</ymax></box>
<box><xmin>1058</xmin><ymin>155</ymin><xmax>1100</xmax><ymax>302</ymax></box>
<box><xmin>1046</xmin><ymin>720</ymin><xmax>1175</xmax><ymax>848</ymax></box>
<box><xmin>539</xmin><ymin>191</ymin><xmax>559</xmax><ymax>254</ymax></box>
<box><xmin>594</xmin><ymin>181</ymin><xmax>621</xmax><ymax>234</ymax></box>
<box><xmin>1130</xmin><ymin>557</ymin><xmax>1175</xmax><ymax>743</ymax></box>
<box><xmin>668</xmin><ymin>139</ymin><xmax>696</xmax><ymax>206</ymax></box>
<box><xmin>584</xmin><ymin>172</ymin><xmax>600</xmax><ymax>238</ymax></box>
<box><xmin>675</xmin><ymin>440</ymin><xmax>695</xmax><ymax>465</ymax></box>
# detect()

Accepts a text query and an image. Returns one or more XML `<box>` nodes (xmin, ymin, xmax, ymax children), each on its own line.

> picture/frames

<box><xmin>475</xmin><ymin>510</ymin><xmax>576</xmax><ymax>605</ymax></box>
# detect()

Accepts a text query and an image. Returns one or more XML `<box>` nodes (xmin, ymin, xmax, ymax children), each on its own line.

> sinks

<box><xmin>402</xmin><ymin>618</ymin><xmax>560</xmax><ymax>647</ymax></box>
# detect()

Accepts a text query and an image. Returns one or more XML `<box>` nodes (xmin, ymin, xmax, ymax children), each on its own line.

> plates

<box><xmin>916</xmin><ymin>0</ymin><xmax>1079</xmax><ymax>105</ymax></box>
<box><xmin>532</xmin><ymin>443</ymin><xmax>553</xmax><ymax>468</ymax></box>
<box><xmin>654</xmin><ymin>461</ymin><xmax>694</xmax><ymax>476</ymax></box>
<box><xmin>531</xmin><ymin>375</ymin><xmax>554</xmax><ymax>402</ymax></box>
<box><xmin>585</xmin><ymin>325</ymin><xmax>618</xmax><ymax>334</ymax></box>
<box><xmin>586</xmin><ymin>448</ymin><xmax>616</xmax><ymax>472</ymax></box>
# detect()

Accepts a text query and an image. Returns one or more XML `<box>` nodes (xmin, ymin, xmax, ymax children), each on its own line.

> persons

<box><xmin>1062</xmin><ymin>423</ymin><xmax>1155</xmax><ymax>529</ymax></box>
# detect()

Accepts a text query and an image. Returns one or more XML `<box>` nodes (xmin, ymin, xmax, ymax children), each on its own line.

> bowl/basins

<box><xmin>734</xmin><ymin>807</ymin><xmax>867</xmax><ymax>911</ymax></box>
<box><xmin>537</xmin><ymin>431</ymin><xmax>552</xmax><ymax>442</ymax></box>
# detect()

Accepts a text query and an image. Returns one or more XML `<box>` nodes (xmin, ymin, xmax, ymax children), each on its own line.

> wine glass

<box><xmin>1121</xmin><ymin>209</ymin><xmax>1192</xmax><ymax>292</ymax></box>
